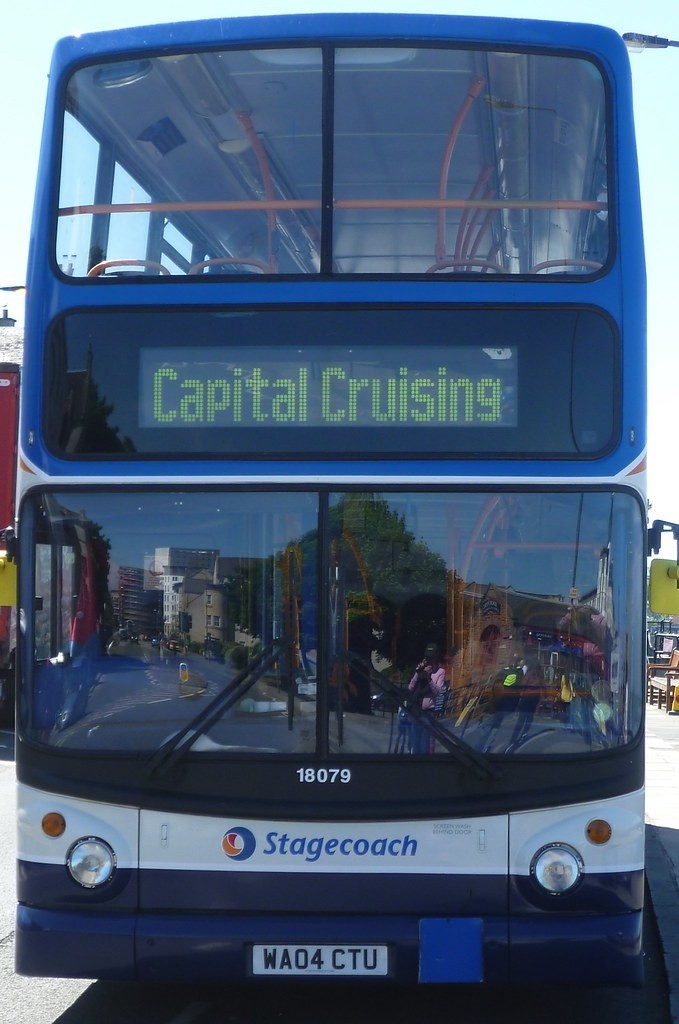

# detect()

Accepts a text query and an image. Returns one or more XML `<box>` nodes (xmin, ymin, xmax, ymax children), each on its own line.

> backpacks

<box><xmin>399</xmin><ymin>709</ymin><xmax>410</xmax><ymax>721</ymax></box>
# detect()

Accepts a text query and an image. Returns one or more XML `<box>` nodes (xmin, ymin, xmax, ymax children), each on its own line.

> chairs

<box><xmin>88</xmin><ymin>258</ymin><xmax>173</xmax><ymax>275</ymax></box>
<box><xmin>527</xmin><ymin>258</ymin><xmax>603</xmax><ymax>275</ymax></box>
<box><xmin>428</xmin><ymin>260</ymin><xmax>510</xmax><ymax>274</ymax></box>
<box><xmin>647</xmin><ymin>646</ymin><xmax>679</xmax><ymax>713</ymax></box>
<box><xmin>190</xmin><ymin>257</ymin><xmax>272</xmax><ymax>274</ymax></box>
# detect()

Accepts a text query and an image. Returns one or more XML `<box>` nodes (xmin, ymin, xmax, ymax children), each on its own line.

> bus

<box><xmin>0</xmin><ymin>11</ymin><xmax>678</xmax><ymax>985</ymax></box>
<box><xmin>31</xmin><ymin>521</ymin><xmax>112</xmax><ymax>729</ymax></box>
<box><xmin>0</xmin><ymin>361</ymin><xmax>21</xmax><ymax>731</ymax></box>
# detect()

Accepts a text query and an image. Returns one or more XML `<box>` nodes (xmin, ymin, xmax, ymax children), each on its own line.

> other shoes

<box><xmin>570</xmin><ymin>724</ymin><xmax>575</xmax><ymax>731</ymax></box>
<box><xmin>561</xmin><ymin>724</ymin><xmax>566</xmax><ymax>730</ymax></box>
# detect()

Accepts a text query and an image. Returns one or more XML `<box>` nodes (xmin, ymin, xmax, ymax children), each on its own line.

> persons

<box><xmin>474</xmin><ymin>658</ymin><xmax>548</xmax><ymax>754</ymax></box>
<box><xmin>560</xmin><ymin>669</ymin><xmax>574</xmax><ymax>724</ymax></box>
<box><xmin>394</xmin><ymin>642</ymin><xmax>450</xmax><ymax>751</ymax></box>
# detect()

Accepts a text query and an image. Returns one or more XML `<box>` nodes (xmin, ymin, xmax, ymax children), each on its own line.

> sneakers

<box><xmin>505</xmin><ymin>744</ymin><xmax>514</xmax><ymax>755</ymax></box>
<box><xmin>517</xmin><ymin>733</ymin><xmax>528</xmax><ymax>744</ymax></box>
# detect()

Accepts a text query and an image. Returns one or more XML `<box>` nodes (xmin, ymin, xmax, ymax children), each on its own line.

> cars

<box><xmin>168</xmin><ymin>639</ymin><xmax>180</xmax><ymax>651</ymax></box>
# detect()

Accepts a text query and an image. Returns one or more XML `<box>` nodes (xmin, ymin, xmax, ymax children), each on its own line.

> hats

<box><xmin>424</xmin><ymin>643</ymin><xmax>438</xmax><ymax>657</ymax></box>
